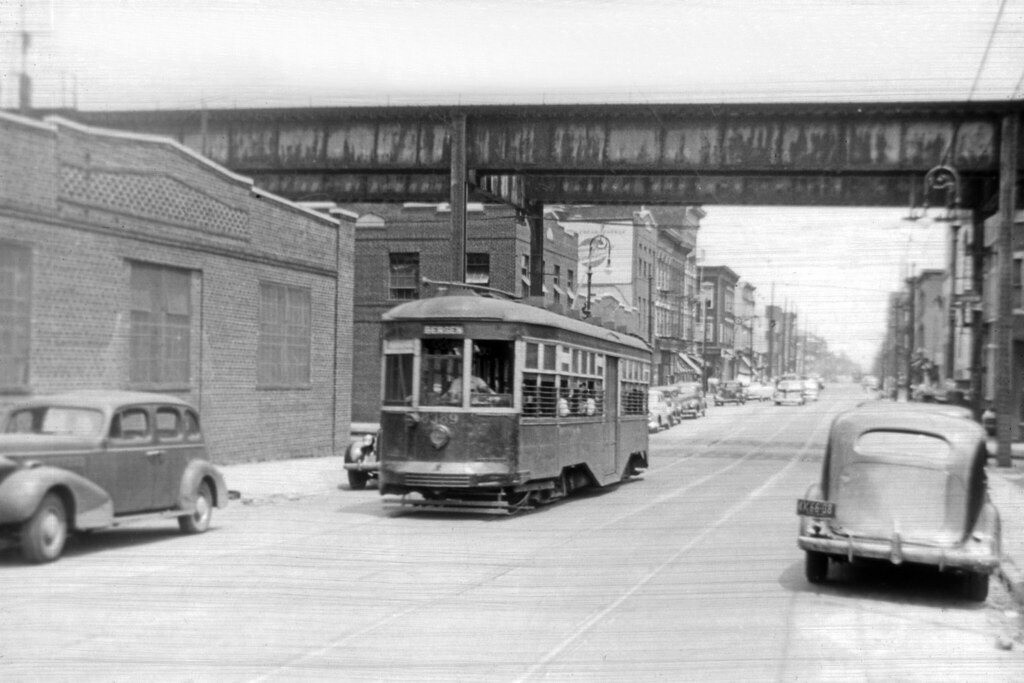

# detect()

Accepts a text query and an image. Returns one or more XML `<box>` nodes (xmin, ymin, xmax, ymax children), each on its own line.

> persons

<box><xmin>523</xmin><ymin>382</ymin><xmax>602</xmax><ymax>417</ymax></box>
<box><xmin>441</xmin><ymin>361</ymin><xmax>501</xmax><ymax>403</ymax></box>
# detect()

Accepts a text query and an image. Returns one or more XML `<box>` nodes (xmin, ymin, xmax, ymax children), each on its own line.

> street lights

<box><xmin>902</xmin><ymin>165</ymin><xmax>965</xmax><ymax>381</ymax></box>
<box><xmin>583</xmin><ymin>234</ymin><xmax>613</xmax><ymax>323</ymax></box>
<box><xmin>731</xmin><ymin>316</ymin><xmax>762</xmax><ymax>378</ymax></box>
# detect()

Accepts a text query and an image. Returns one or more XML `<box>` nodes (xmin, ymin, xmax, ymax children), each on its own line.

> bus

<box><xmin>374</xmin><ymin>295</ymin><xmax>651</xmax><ymax>517</ymax></box>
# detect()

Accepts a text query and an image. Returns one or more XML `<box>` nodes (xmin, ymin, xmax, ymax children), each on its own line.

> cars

<box><xmin>0</xmin><ymin>391</ymin><xmax>230</xmax><ymax>564</ymax></box>
<box><xmin>345</xmin><ymin>433</ymin><xmax>381</xmax><ymax>490</ymax></box>
<box><xmin>676</xmin><ymin>382</ymin><xmax>706</xmax><ymax>418</ymax></box>
<box><xmin>772</xmin><ymin>373</ymin><xmax>806</xmax><ymax>407</ymax></box>
<box><xmin>714</xmin><ymin>381</ymin><xmax>747</xmax><ymax>405</ymax></box>
<box><xmin>862</xmin><ymin>375</ymin><xmax>881</xmax><ymax>390</ymax></box>
<box><xmin>793</xmin><ymin>400</ymin><xmax>1001</xmax><ymax>602</ymax></box>
<box><xmin>665</xmin><ymin>393</ymin><xmax>683</xmax><ymax>426</ymax></box>
<box><xmin>802</xmin><ymin>376</ymin><xmax>820</xmax><ymax>401</ymax></box>
<box><xmin>811</xmin><ymin>374</ymin><xmax>825</xmax><ymax>390</ymax></box>
<box><xmin>743</xmin><ymin>380</ymin><xmax>772</xmax><ymax>401</ymax></box>
<box><xmin>648</xmin><ymin>391</ymin><xmax>674</xmax><ymax>431</ymax></box>
